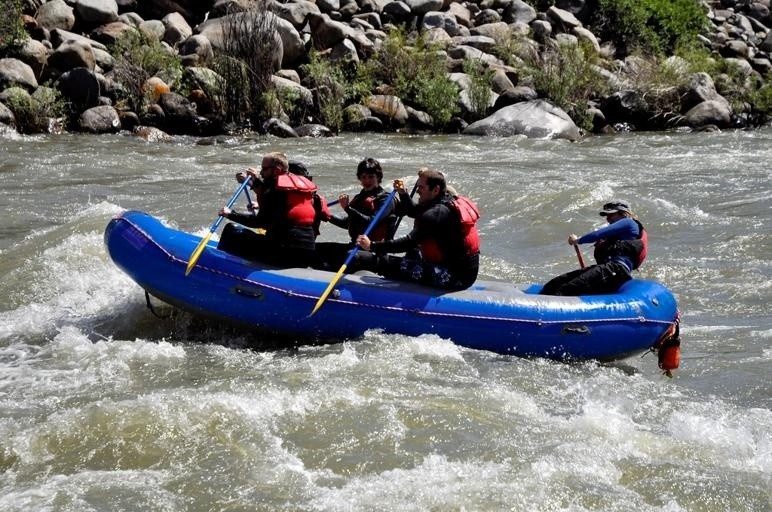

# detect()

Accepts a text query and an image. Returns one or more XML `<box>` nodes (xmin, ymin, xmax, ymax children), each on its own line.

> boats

<box><xmin>104</xmin><ymin>211</ymin><xmax>678</xmax><ymax>363</ymax></box>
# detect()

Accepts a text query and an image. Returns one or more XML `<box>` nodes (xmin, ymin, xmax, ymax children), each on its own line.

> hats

<box><xmin>599</xmin><ymin>197</ymin><xmax>632</xmax><ymax>217</ymax></box>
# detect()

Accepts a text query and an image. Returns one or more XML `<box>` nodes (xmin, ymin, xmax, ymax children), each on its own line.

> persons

<box><xmin>236</xmin><ymin>160</ymin><xmax>322</xmax><ymax>244</ymax></box>
<box><xmin>309</xmin><ymin>157</ymin><xmax>398</xmax><ymax>273</ymax></box>
<box><xmin>345</xmin><ymin>166</ymin><xmax>479</xmax><ymax>292</ymax></box>
<box><xmin>216</xmin><ymin>151</ymin><xmax>319</xmax><ymax>268</ymax></box>
<box><xmin>538</xmin><ymin>199</ymin><xmax>646</xmax><ymax>297</ymax></box>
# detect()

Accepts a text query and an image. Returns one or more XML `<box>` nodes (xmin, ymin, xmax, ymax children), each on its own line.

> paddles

<box><xmin>242</xmin><ymin>180</ymin><xmax>267</xmax><ymax>235</ymax></box>
<box><xmin>185</xmin><ymin>175</ymin><xmax>251</xmax><ymax>277</ymax></box>
<box><xmin>308</xmin><ymin>189</ymin><xmax>397</xmax><ymax>319</ymax></box>
